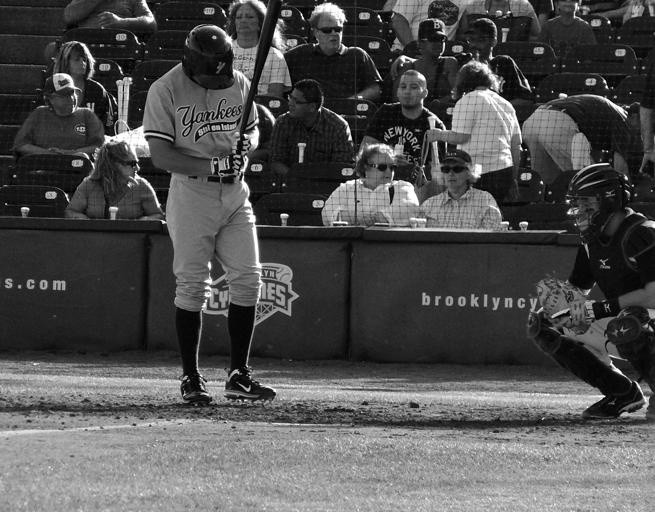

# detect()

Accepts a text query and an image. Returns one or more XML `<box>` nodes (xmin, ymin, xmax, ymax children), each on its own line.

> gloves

<box><xmin>209</xmin><ymin>154</ymin><xmax>249</xmax><ymax>176</ymax></box>
<box><xmin>536</xmin><ymin>277</ymin><xmax>594</xmax><ymax>338</ymax></box>
<box><xmin>231</xmin><ymin>128</ymin><xmax>250</xmax><ymax>157</ymax></box>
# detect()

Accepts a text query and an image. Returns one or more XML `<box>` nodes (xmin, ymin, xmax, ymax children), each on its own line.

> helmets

<box><xmin>564</xmin><ymin>160</ymin><xmax>635</xmax><ymax>244</ymax></box>
<box><xmin>181</xmin><ymin>22</ymin><xmax>237</xmax><ymax>91</ymax></box>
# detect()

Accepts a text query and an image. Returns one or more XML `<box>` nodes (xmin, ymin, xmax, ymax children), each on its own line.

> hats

<box><xmin>442</xmin><ymin>148</ymin><xmax>472</xmax><ymax>165</ymax></box>
<box><xmin>417</xmin><ymin>17</ymin><xmax>448</xmax><ymax>41</ymax></box>
<box><xmin>43</xmin><ymin>71</ymin><xmax>82</xmax><ymax>96</ymax></box>
<box><xmin>459</xmin><ymin>18</ymin><xmax>498</xmax><ymax>42</ymax></box>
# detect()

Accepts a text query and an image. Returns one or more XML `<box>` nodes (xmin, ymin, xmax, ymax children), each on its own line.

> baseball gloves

<box><xmin>538</xmin><ymin>277</ymin><xmax>590</xmax><ymax>333</ymax></box>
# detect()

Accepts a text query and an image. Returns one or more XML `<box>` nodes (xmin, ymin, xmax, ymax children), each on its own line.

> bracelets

<box><xmin>590</xmin><ymin>299</ymin><xmax>619</xmax><ymax>320</ymax></box>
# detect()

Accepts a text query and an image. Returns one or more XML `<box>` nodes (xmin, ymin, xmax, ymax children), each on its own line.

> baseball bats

<box><xmin>235</xmin><ymin>0</ymin><xmax>283</xmax><ymax>153</ymax></box>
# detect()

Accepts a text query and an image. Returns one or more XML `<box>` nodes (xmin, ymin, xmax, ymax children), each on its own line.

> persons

<box><xmin>145</xmin><ymin>24</ymin><xmax>276</xmax><ymax>403</ymax></box>
<box><xmin>63</xmin><ymin>137</ymin><xmax>164</xmax><ymax>223</ymax></box>
<box><xmin>13</xmin><ymin>74</ymin><xmax>105</xmax><ymax>164</ymax></box>
<box><xmin>62</xmin><ymin>0</ymin><xmax>158</xmax><ymax>37</ymax></box>
<box><xmin>526</xmin><ymin>161</ymin><xmax>655</xmax><ymax>422</ymax></box>
<box><xmin>51</xmin><ymin>41</ymin><xmax>112</xmax><ymax>135</ymax></box>
<box><xmin>219</xmin><ymin>0</ymin><xmax>655</xmax><ymax>232</ymax></box>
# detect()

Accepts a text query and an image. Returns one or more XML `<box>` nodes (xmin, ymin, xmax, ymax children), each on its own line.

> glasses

<box><xmin>287</xmin><ymin>93</ymin><xmax>315</xmax><ymax>104</ymax></box>
<box><xmin>117</xmin><ymin>158</ymin><xmax>140</xmax><ymax>167</ymax></box>
<box><xmin>314</xmin><ymin>25</ymin><xmax>344</xmax><ymax>34</ymax></box>
<box><xmin>440</xmin><ymin>165</ymin><xmax>469</xmax><ymax>174</ymax></box>
<box><xmin>366</xmin><ymin>161</ymin><xmax>399</xmax><ymax>172</ymax></box>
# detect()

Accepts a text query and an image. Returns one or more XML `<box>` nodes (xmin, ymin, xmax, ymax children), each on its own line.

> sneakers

<box><xmin>222</xmin><ymin>368</ymin><xmax>278</xmax><ymax>404</ymax></box>
<box><xmin>176</xmin><ymin>372</ymin><xmax>215</xmax><ymax>410</ymax></box>
<box><xmin>579</xmin><ymin>379</ymin><xmax>646</xmax><ymax>422</ymax></box>
